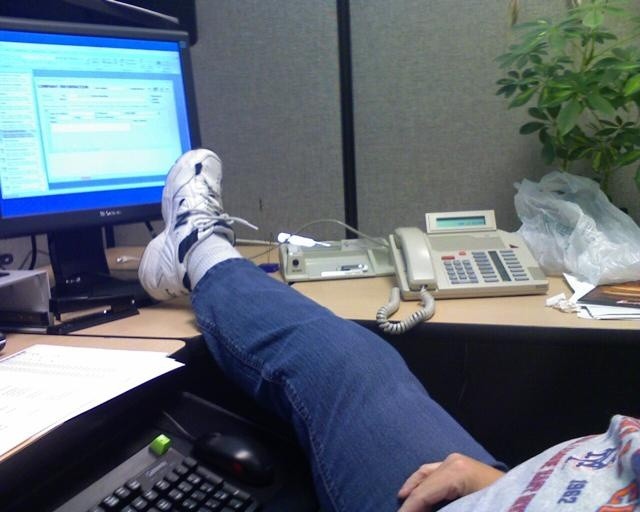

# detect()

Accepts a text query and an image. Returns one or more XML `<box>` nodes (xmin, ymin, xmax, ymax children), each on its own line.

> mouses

<box><xmin>193</xmin><ymin>431</ymin><xmax>273</xmax><ymax>484</ymax></box>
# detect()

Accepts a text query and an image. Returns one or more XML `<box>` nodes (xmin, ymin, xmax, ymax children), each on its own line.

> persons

<box><xmin>135</xmin><ymin>146</ymin><xmax>639</xmax><ymax>512</ymax></box>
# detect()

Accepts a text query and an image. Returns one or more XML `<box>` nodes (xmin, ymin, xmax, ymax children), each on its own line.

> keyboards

<box><xmin>51</xmin><ymin>433</ymin><xmax>262</xmax><ymax>512</ymax></box>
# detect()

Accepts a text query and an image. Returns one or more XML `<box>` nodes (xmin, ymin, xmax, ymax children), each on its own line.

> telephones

<box><xmin>388</xmin><ymin>209</ymin><xmax>550</xmax><ymax>300</ymax></box>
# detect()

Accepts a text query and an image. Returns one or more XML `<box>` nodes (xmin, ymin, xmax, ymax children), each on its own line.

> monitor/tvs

<box><xmin>0</xmin><ymin>17</ymin><xmax>202</xmax><ymax>314</ymax></box>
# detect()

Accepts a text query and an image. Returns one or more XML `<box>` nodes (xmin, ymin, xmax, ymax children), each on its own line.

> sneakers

<box><xmin>136</xmin><ymin>147</ymin><xmax>237</xmax><ymax>302</ymax></box>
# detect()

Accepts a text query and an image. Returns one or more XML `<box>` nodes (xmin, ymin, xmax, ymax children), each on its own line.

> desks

<box><xmin>0</xmin><ymin>247</ymin><xmax>639</xmax><ymax>464</ymax></box>
